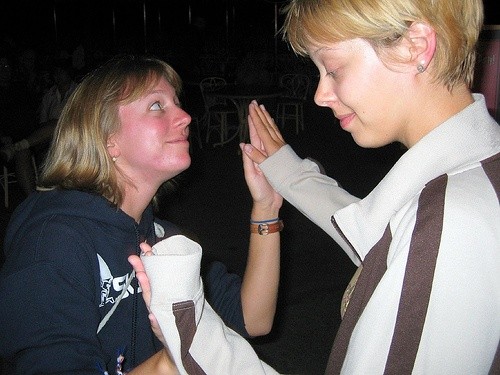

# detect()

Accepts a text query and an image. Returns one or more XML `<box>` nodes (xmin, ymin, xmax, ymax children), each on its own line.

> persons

<box><xmin>127</xmin><ymin>0</ymin><xmax>500</xmax><ymax>375</ymax></box>
<box><xmin>0</xmin><ymin>54</ymin><xmax>283</xmax><ymax>375</ymax></box>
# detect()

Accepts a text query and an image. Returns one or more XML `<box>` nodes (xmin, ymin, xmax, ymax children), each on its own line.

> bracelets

<box><xmin>250</xmin><ymin>221</ymin><xmax>284</xmax><ymax>235</ymax></box>
<box><xmin>250</xmin><ymin>218</ymin><xmax>279</xmax><ymax>223</ymax></box>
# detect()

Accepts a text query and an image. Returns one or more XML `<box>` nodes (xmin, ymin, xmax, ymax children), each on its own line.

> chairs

<box><xmin>0</xmin><ymin>53</ymin><xmax>310</xmax><ymax>209</ymax></box>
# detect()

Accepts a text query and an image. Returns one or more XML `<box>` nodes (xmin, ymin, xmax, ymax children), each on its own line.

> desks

<box><xmin>206</xmin><ymin>84</ymin><xmax>293</xmax><ymax>155</ymax></box>
